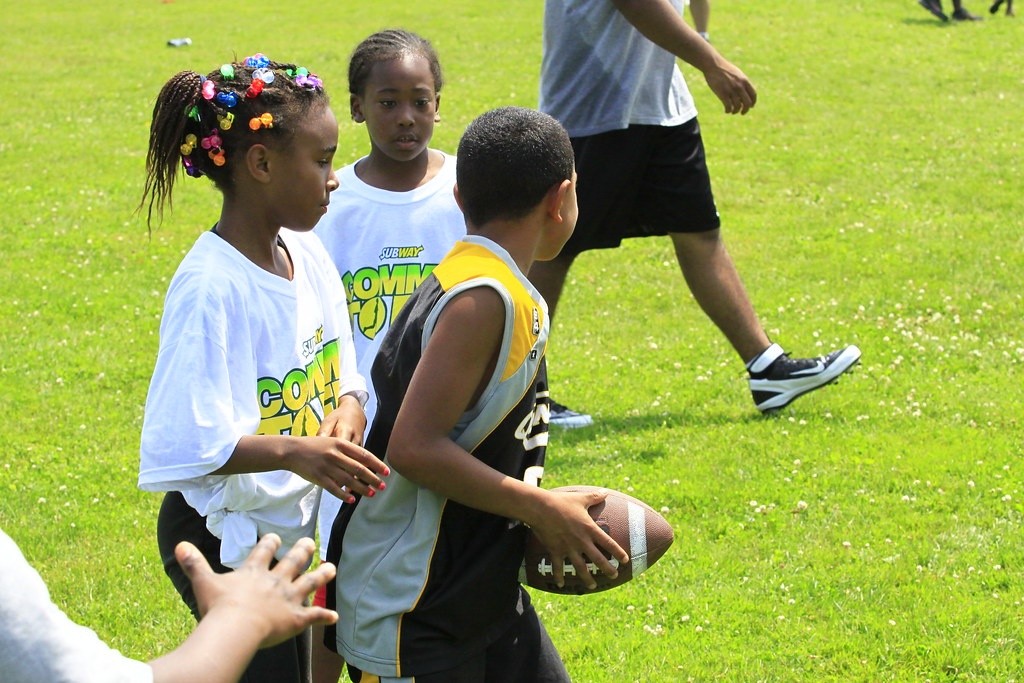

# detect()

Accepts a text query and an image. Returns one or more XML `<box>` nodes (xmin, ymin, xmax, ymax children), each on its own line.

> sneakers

<box><xmin>549</xmin><ymin>399</ymin><xmax>592</xmax><ymax>430</ymax></box>
<box><xmin>747</xmin><ymin>342</ymin><xmax>861</xmax><ymax>413</ymax></box>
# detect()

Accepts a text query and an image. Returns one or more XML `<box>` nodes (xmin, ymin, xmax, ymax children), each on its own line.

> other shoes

<box><xmin>912</xmin><ymin>0</ymin><xmax>1014</xmax><ymax>20</ymax></box>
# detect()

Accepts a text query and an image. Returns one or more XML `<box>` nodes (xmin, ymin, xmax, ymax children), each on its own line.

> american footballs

<box><xmin>516</xmin><ymin>485</ymin><xmax>675</xmax><ymax>596</ymax></box>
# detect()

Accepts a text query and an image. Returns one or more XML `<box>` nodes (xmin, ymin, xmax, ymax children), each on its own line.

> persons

<box><xmin>536</xmin><ymin>0</ymin><xmax>865</xmax><ymax>426</ymax></box>
<box><xmin>319</xmin><ymin>104</ymin><xmax>629</xmax><ymax>683</ymax></box>
<box><xmin>919</xmin><ymin>0</ymin><xmax>982</xmax><ymax>24</ymax></box>
<box><xmin>140</xmin><ymin>53</ymin><xmax>393</xmax><ymax>683</ymax></box>
<box><xmin>990</xmin><ymin>0</ymin><xmax>1018</xmax><ymax>17</ymax></box>
<box><xmin>309</xmin><ymin>30</ymin><xmax>467</xmax><ymax>683</ymax></box>
<box><xmin>0</xmin><ymin>525</ymin><xmax>340</xmax><ymax>683</ymax></box>
